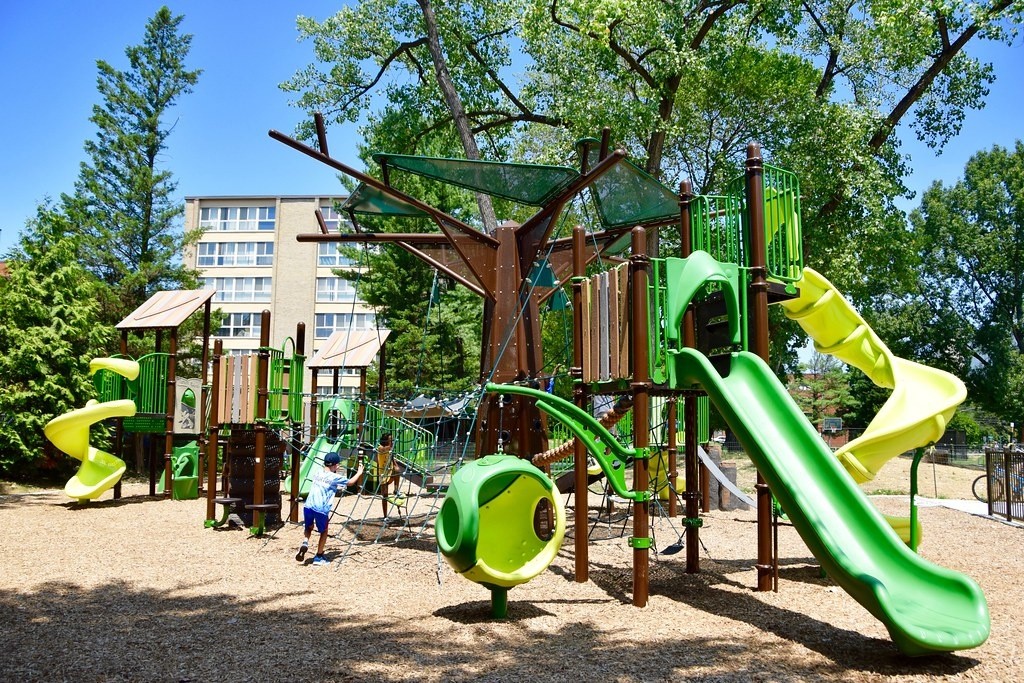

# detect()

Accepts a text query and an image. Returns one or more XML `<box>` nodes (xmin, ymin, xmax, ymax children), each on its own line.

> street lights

<box><xmin>1010</xmin><ymin>422</ymin><xmax>1014</xmax><ymax>450</ymax></box>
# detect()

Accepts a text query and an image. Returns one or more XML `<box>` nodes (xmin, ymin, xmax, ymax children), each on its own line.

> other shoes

<box><xmin>296</xmin><ymin>541</ymin><xmax>308</xmax><ymax>561</ymax></box>
<box><xmin>393</xmin><ymin>490</ymin><xmax>401</xmax><ymax>496</ymax></box>
<box><xmin>313</xmin><ymin>555</ymin><xmax>330</xmax><ymax>565</ymax></box>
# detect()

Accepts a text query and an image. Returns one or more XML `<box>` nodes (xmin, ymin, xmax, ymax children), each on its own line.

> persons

<box><xmin>377</xmin><ymin>433</ymin><xmax>406</xmax><ymax>522</ymax></box>
<box><xmin>295</xmin><ymin>452</ymin><xmax>365</xmax><ymax>565</ymax></box>
<box><xmin>593</xmin><ymin>395</ymin><xmax>620</xmax><ymax>512</ymax></box>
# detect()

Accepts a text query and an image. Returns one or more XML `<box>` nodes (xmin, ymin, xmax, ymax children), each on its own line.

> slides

<box><xmin>677</xmin><ymin>345</ymin><xmax>992</xmax><ymax>649</ymax></box>
<box><xmin>648</xmin><ymin>448</ymin><xmax>686</xmax><ymax>501</ymax></box>
<box><xmin>782</xmin><ymin>265</ymin><xmax>970</xmax><ymax>546</ymax></box>
<box><xmin>44</xmin><ymin>398</ymin><xmax>137</xmax><ymax>500</ymax></box>
<box><xmin>284</xmin><ymin>433</ymin><xmax>347</xmax><ymax>497</ymax></box>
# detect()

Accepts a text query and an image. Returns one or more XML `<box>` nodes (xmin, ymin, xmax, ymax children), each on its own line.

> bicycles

<box><xmin>972</xmin><ymin>460</ymin><xmax>1024</xmax><ymax>503</ymax></box>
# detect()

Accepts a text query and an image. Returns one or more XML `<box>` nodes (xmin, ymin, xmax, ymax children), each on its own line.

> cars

<box><xmin>329</xmin><ymin>419</ymin><xmax>359</xmax><ymax>432</ymax></box>
<box><xmin>711</xmin><ymin>436</ymin><xmax>726</xmax><ymax>443</ymax></box>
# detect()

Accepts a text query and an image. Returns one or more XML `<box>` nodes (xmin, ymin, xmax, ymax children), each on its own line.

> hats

<box><xmin>324</xmin><ymin>452</ymin><xmax>340</xmax><ymax>466</ymax></box>
